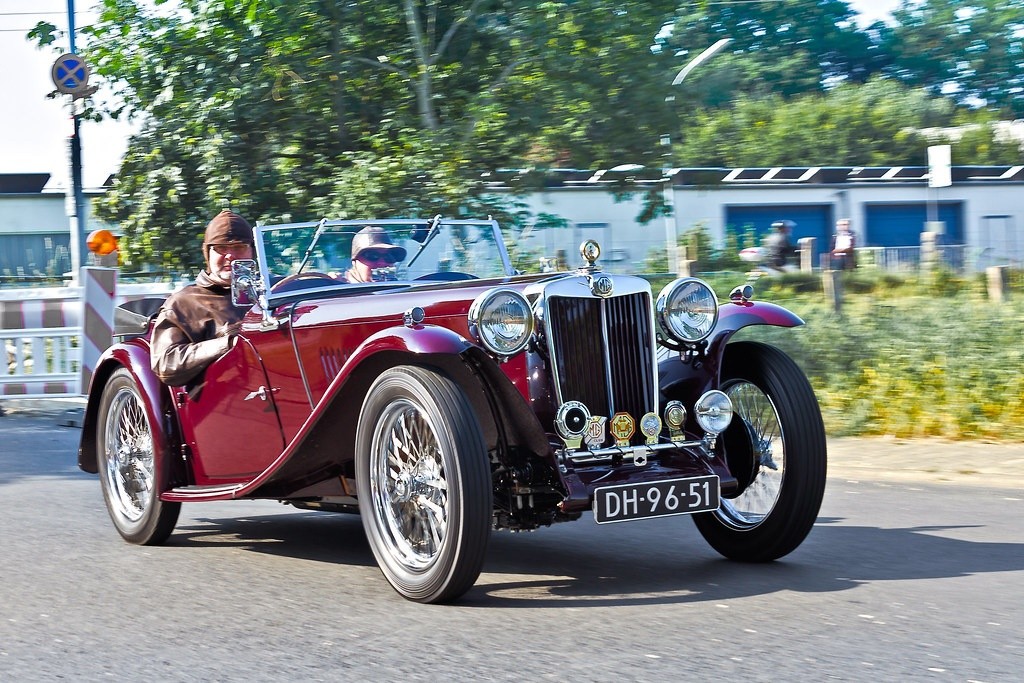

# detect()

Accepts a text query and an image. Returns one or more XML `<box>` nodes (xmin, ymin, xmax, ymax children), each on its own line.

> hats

<box><xmin>351</xmin><ymin>225</ymin><xmax>408</xmax><ymax>263</ymax></box>
<box><xmin>202</xmin><ymin>209</ymin><xmax>255</xmax><ymax>246</ymax></box>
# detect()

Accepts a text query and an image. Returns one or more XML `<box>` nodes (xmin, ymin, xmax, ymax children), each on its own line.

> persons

<box><xmin>150</xmin><ymin>211</ymin><xmax>258</xmax><ymax>386</ymax></box>
<box><xmin>328</xmin><ymin>227</ymin><xmax>407</xmax><ymax>285</ymax></box>
<box><xmin>766</xmin><ymin>220</ymin><xmax>799</xmax><ymax>273</ymax></box>
<box><xmin>832</xmin><ymin>220</ymin><xmax>854</xmax><ymax>271</ymax></box>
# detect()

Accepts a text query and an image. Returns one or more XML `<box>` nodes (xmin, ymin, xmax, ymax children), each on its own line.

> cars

<box><xmin>76</xmin><ymin>217</ymin><xmax>832</xmax><ymax>607</ymax></box>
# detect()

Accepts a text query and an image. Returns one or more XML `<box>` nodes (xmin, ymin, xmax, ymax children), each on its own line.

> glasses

<box><xmin>359</xmin><ymin>250</ymin><xmax>398</xmax><ymax>264</ymax></box>
<box><xmin>210</xmin><ymin>245</ymin><xmax>252</xmax><ymax>256</ymax></box>
